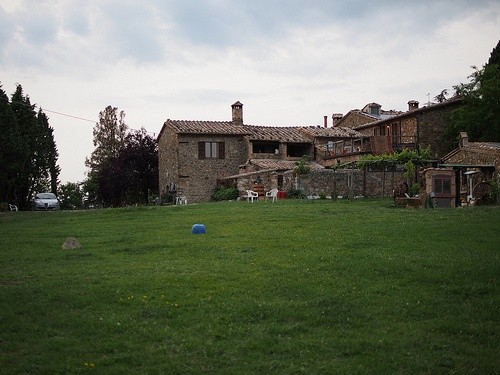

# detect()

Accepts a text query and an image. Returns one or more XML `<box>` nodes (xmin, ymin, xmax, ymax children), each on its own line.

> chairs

<box><xmin>246</xmin><ymin>189</ymin><xmax>258</xmax><ymax>204</ymax></box>
<box><xmin>9</xmin><ymin>204</ymin><xmax>18</xmax><ymax>212</ymax></box>
<box><xmin>265</xmin><ymin>189</ymin><xmax>279</xmax><ymax>203</ymax></box>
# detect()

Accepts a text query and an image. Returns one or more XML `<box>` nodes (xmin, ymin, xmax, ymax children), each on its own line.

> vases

<box><xmin>278</xmin><ymin>191</ymin><xmax>286</xmax><ymax>198</ymax></box>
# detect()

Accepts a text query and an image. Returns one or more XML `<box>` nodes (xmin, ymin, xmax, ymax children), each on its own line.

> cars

<box><xmin>34</xmin><ymin>192</ymin><xmax>60</xmax><ymax>211</ymax></box>
